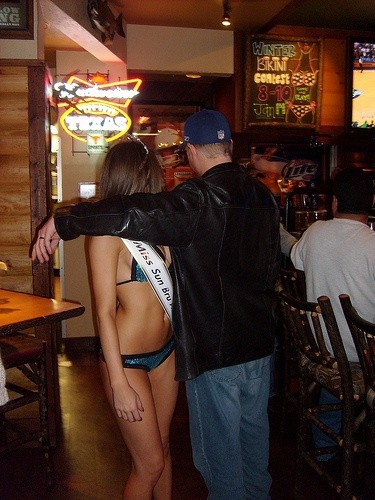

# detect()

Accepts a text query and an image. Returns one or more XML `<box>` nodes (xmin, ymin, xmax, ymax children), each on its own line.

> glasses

<box><xmin>126</xmin><ymin>134</ymin><xmax>149</xmax><ymax>173</ymax></box>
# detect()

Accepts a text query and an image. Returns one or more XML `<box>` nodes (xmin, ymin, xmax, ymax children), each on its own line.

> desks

<box><xmin>0</xmin><ymin>288</ymin><xmax>85</xmax><ymax>338</ymax></box>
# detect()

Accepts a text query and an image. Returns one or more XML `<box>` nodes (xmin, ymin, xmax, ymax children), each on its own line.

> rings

<box><xmin>40</xmin><ymin>237</ymin><xmax>44</xmax><ymax>239</ymax></box>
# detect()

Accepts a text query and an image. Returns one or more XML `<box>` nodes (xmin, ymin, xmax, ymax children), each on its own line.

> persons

<box><xmin>85</xmin><ymin>141</ymin><xmax>180</xmax><ymax>500</ymax></box>
<box><xmin>32</xmin><ymin>109</ymin><xmax>280</xmax><ymax>500</ymax></box>
<box><xmin>290</xmin><ymin>169</ymin><xmax>375</xmax><ymax>464</ymax></box>
<box><xmin>247</xmin><ymin>163</ymin><xmax>298</xmax><ymax>413</ymax></box>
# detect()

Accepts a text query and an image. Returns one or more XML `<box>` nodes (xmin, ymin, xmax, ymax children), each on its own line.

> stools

<box><xmin>0</xmin><ymin>331</ymin><xmax>56</xmax><ymax>485</ymax></box>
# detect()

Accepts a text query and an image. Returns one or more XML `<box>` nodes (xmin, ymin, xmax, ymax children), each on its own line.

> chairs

<box><xmin>289</xmin><ymin>186</ymin><xmax>333</xmax><ymax>230</ymax></box>
<box><xmin>339</xmin><ymin>294</ymin><xmax>375</xmax><ymax>500</ymax></box>
<box><xmin>274</xmin><ymin>273</ymin><xmax>366</xmax><ymax>500</ymax></box>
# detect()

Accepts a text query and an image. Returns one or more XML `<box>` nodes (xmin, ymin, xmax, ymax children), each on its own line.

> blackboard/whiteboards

<box><xmin>244</xmin><ymin>35</ymin><xmax>324</xmax><ymax>127</ymax></box>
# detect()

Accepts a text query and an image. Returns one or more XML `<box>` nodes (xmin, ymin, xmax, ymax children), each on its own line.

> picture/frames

<box><xmin>0</xmin><ymin>0</ymin><xmax>34</xmax><ymax>39</ymax></box>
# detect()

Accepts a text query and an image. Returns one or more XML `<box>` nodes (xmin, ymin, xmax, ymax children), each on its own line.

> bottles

<box><xmin>284</xmin><ymin>198</ymin><xmax>292</xmax><ymax>233</ymax></box>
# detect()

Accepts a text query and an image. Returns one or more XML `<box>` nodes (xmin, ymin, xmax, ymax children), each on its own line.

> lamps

<box><xmin>220</xmin><ymin>4</ymin><xmax>233</xmax><ymax>28</ymax></box>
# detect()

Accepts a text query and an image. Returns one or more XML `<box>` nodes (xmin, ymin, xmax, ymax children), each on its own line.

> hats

<box><xmin>174</xmin><ymin>109</ymin><xmax>232</xmax><ymax>149</ymax></box>
